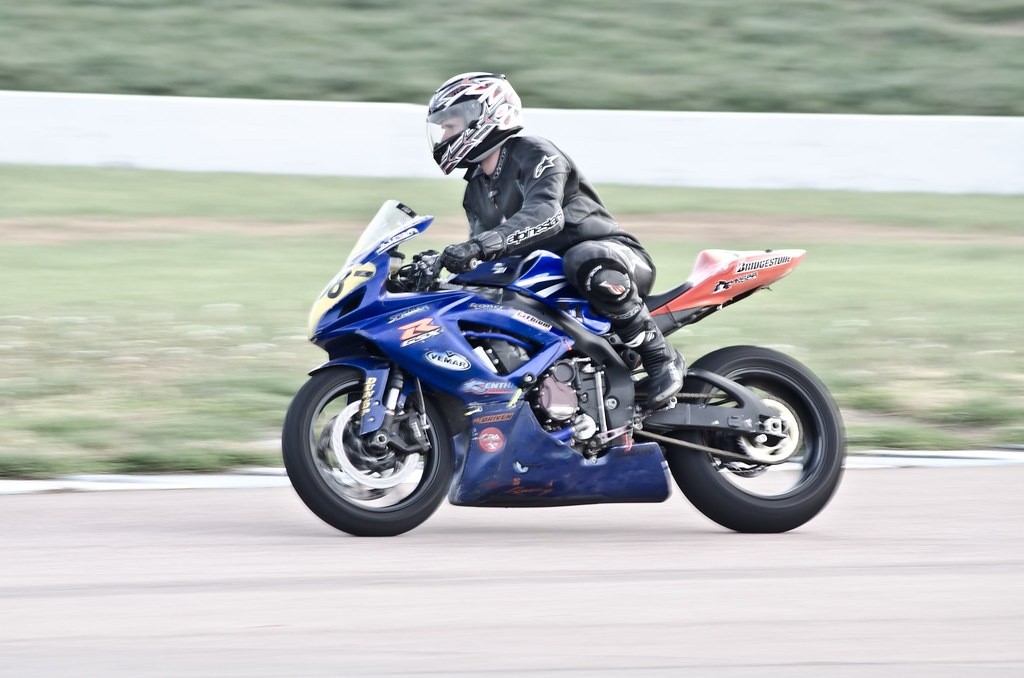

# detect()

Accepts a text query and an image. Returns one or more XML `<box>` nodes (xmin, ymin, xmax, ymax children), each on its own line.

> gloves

<box><xmin>441</xmin><ymin>240</ymin><xmax>482</xmax><ymax>274</ymax></box>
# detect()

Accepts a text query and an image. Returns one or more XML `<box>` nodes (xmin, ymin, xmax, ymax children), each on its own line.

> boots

<box><xmin>618</xmin><ymin>302</ymin><xmax>687</xmax><ymax>412</ymax></box>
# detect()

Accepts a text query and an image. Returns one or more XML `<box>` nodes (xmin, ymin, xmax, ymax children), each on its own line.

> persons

<box><xmin>426</xmin><ymin>72</ymin><xmax>688</xmax><ymax>409</ymax></box>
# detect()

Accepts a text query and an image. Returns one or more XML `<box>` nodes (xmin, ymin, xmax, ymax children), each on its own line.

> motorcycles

<box><xmin>281</xmin><ymin>199</ymin><xmax>853</xmax><ymax>539</ymax></box>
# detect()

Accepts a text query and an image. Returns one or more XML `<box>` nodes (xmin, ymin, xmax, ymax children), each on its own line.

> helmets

<box><xmin>425</xmin><ymin>72</ymin><xmax>523</xmax><ymax>175</ymax></box>
